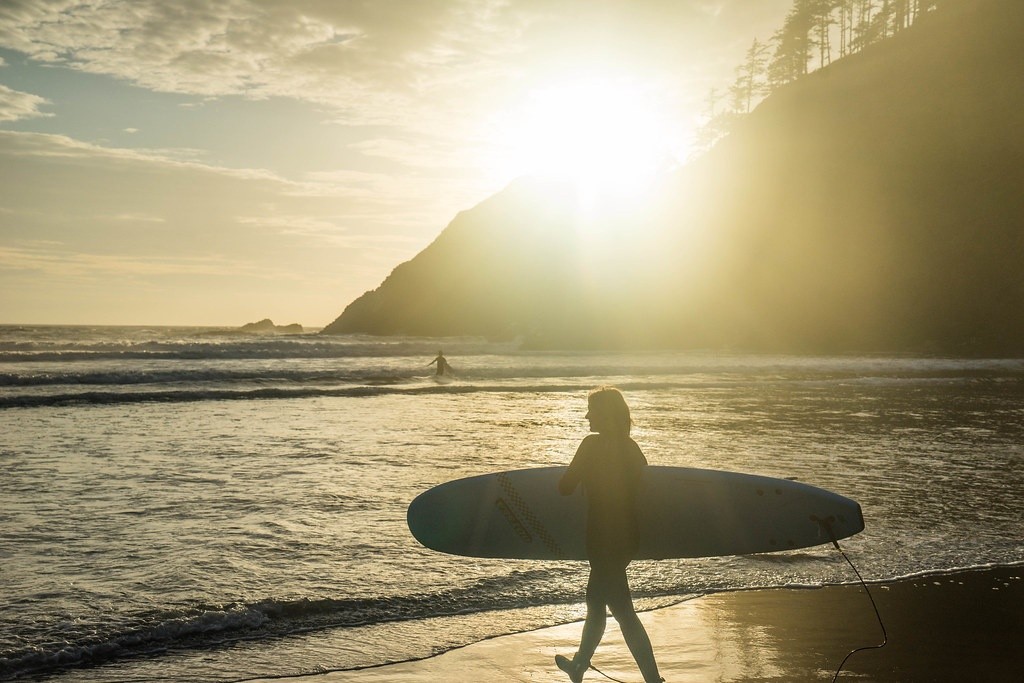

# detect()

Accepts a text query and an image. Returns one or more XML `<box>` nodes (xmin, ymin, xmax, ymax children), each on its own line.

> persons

<box><xmin>426</xmin><ymin>351</ymin><xmax>449</xmax><ymax>376</ymax></box>
<box><xmin>554</xmin><ymin>389</ymin><xmax>667</xmax><ymax>683</ymax></box>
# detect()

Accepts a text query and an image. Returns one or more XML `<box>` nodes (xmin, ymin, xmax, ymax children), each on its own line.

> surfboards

<box><xmin>405</xmin><ymin>463</ymin><xmax>864</xmax><ymax>562</ymax></box>
